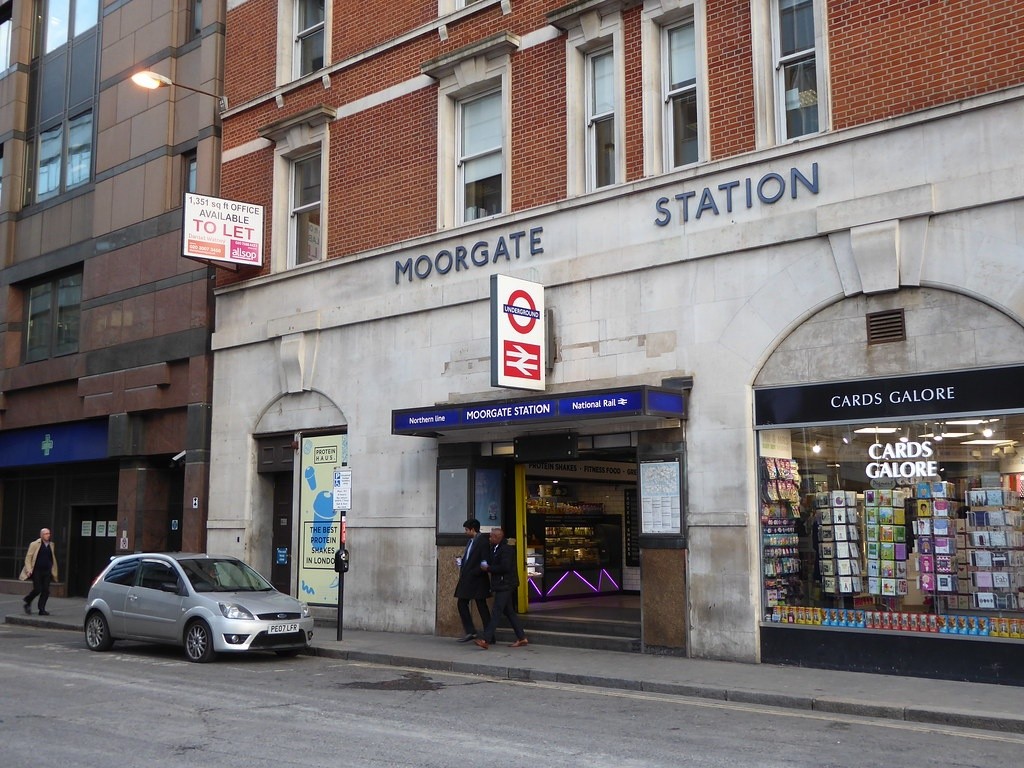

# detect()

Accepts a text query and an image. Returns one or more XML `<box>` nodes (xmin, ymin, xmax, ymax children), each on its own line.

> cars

<box><xmin>84</xmin><ymin>553</ymin><xmax>314</xmax><ymax>663</ymax></box>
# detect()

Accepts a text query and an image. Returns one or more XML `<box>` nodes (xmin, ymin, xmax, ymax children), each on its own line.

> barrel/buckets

<box><xmin>539</xmin><ymin>485</ymin><xmax>552</xmax><ymax>497</ymax></box>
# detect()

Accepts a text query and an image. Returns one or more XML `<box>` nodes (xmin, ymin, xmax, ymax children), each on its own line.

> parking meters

<box><xmin>334</xmin><ymin>549</ymin><xmax>349</xmax><ymax>642</ymax></box>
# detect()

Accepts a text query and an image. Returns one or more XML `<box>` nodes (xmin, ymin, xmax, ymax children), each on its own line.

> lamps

<box><xmin>982</xmin><ymin>419</ymin><xmax>992</xmax><ymax>437</ymax></box>
<box><xmin>992</xmin><ymin>444</ymin><xmax>1016</xmax><ymax>460</ymax></box>
<box><xmin>899</xmin><ymin>426</ymin><xmax>910</xmax><ymax>442</ymax></box>
<box><xmin>933</xmin><ymin>420</ymin><xmax>942</xmax><ymax>440</ymax></box>
<box><xmin>842</xmin><ymin>432</ymin><xmax>857</xmax><ymax>444</ymax></box>
<box><xmin>132</xmin><ymin>70</ymin><xmax>228</xmax><ymax>112</ymax></box>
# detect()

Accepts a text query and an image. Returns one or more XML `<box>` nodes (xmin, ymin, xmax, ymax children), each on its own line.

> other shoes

<box><xmin>39</xmin><ymin>611</ymin><xmax>49</xmax><ymax>615</ymax></box>
<box><xmin>24</xmin><ymin>604</ymin><xmax>30</xmax><ymax>615</ymax></box>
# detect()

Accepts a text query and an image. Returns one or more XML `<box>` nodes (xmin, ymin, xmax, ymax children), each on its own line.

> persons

<box><xmin>917</xmin><ymin>504</ymin><xmax>930</xmax><ymax>516</ymax></box>
<box><xmin>19</xmin><ymin>528</ymin><xmax>58</xmax><ymax>615</ymax></box>
<box><xmin>453</xmin><ymin>518</ymin><xmax>496</xmax><ymax>644</ymax></box>
<box><xmin>918</xmin><ymin>522</ymin><xmax>926</xmax><ymax>534</ymax></box>
<box><xmin>473</xmin><ymin>528</ymin><xmax>528</xmax><ymax>649</ymax></box>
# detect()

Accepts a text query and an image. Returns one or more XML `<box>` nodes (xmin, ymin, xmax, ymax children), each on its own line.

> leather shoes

<box><xmin>487</xmin><ymin>640</ymin><xmax>496</xmax><ymax>644</ymax></box>
<box><xmin>456</xmin><ymin>633</ymin><xmax>479</xmax><ymax>642</ymax></box>
<box><xmin>473</xmin><ymin>639</ymin><xmax>488</xmax><ymax>649</ymax></box>
<box><xmin>509</xmin><ymin>638</ymin><xmax>528</xmax><ymax>647</ymax></box>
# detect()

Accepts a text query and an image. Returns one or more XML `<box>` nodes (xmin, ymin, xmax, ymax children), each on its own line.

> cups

<box><xmin>456</xmin><ymin>556</ymin><xmax>462</xmax><ymax>566</ymax></box>
<box><xmin>481</xmin><ymin>560</ymin><xmax>487</xmax><ymax>565</ymax></box>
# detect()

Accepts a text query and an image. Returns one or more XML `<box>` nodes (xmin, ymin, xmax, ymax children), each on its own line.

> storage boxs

<box><xmin>946</xmin><ymin>518</ymin><xmax>1024</xmax><ymax>611</ymax></box>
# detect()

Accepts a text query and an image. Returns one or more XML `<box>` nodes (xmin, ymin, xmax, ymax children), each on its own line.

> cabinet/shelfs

<box><xmin>527</xmin><ymin>513</ymin><xmax>623</xmax><ymax>602</ymax></box>
